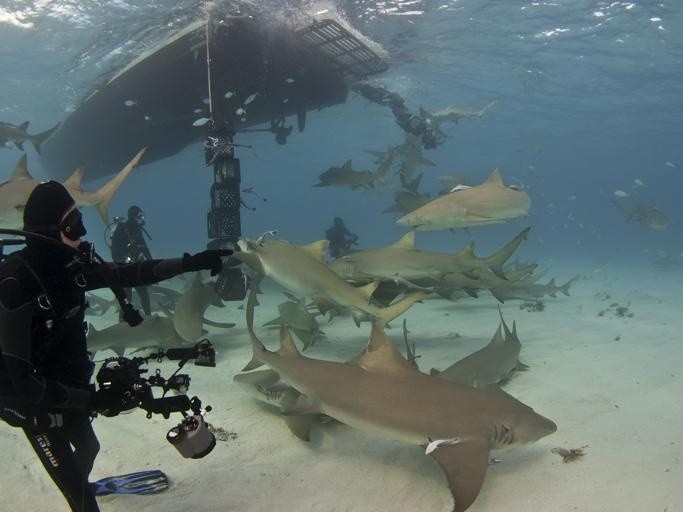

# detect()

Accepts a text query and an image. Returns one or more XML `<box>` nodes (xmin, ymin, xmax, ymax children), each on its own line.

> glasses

<box><xmin>62</xmin><ymin>209</ymin><xmax>87</xmax><ymax>240</ymax></box>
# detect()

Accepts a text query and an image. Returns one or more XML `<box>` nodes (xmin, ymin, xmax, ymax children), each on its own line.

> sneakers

<box><xmin>87</xmin><ymin>481</ymin><xmax>97</xmax><ymax>493</ymax></box>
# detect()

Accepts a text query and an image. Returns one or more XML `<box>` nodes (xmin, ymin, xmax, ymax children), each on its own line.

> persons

<box><xmin>110</xmin><ymin>204</ymin><xmax>154</xmax><ymax>324</ymax></box>
<box><xmin>328</xmin><ymin>216</ymin><xmax>355</xmax><ymax>259</ymax></box>
<box><xmin>1</xmin><ymin>180</ymin><xmax>234</xmax><ymax>512</ymax></box>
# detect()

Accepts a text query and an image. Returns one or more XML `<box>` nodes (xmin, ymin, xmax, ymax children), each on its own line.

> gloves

<box><xmin>184</xmin><ymin>250</ymin><xmax>234</xmax><ymax>277</ymax></box>
<box><xmin>97</xmin><ymin>389</ymin><xmax>139</xmax><ymax>413</ymax></box>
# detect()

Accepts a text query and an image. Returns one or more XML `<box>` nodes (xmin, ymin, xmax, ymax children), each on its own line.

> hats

<box><xmin>128</xmin><ymin>206</ymin><xmax>144</xmax><ymax>229</ymax></box>
<box><xmin>24</xmin><ymin>180</ymin><xmax>82</xmax><ymax>269</ymax></box>
